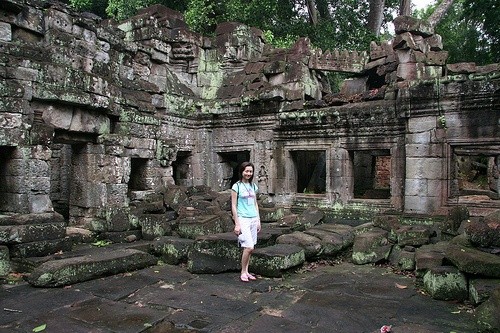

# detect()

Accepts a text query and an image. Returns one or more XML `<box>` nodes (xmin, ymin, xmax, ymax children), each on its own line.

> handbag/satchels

<box><xmin>230</xmin><ymin>194</ymin><xmax>239</xmax><ymax>224</ymax></box>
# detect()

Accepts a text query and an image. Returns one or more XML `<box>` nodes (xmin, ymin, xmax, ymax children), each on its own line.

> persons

<box><xmin>232</xmin><ymin>162</ymin><xmax>261</xmax><ymax>282</ymax></box>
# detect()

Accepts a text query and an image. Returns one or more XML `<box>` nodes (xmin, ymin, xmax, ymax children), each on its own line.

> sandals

<box><xmin>240</xmin><ymin>273</ymin><xmax>256</xmax><ymax>282</ymax></box>
